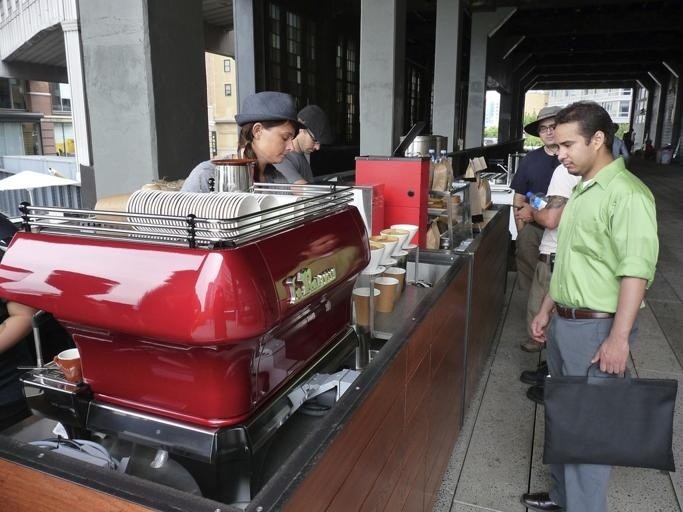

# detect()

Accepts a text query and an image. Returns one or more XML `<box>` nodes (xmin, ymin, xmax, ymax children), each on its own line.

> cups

<box><xmin>372</xmin><ymin>277</ymin><xmax>399</xmax><ymax>314</ymax></box>
<box><xmin>359</xmin><ymin>242</ymin><xmax>384</xmax><ymax>272</ymax></box>
<box><xmin>210</xmin><ymin>158</ymin><xmax>258</xmax><ymax>193</ymax></box>
<box><xmin>353</xmin><ymin>287</ymin><xmax>382</xmax><ymax>328</ymax></box>
<box><xmin>380</xmin><ymin>228</ymin><xmax>409</xmax><ymax>255</ymax></box>
<box><xmin>89</xmin><ymin>188</ymin><xmax>305</xmax><ymax>243</ymax></box>
<box><xmin>385</xmin><ymin>265</ymin><xmax>406</xmax><ymax>300</ymax></box>
<box><xmin>53</xmin><ymin>347</ymin><xmax>81</xmax><ymax>383</ymax></box>
<box><xmin>389</xmin><ymin>221</ymin><xmax>417</xmax><ymax>248</ymax></box>
<box><xmin>370</xmin><ymin>234</ymin><xmax>398</xmax><ymax>265</ymax></box>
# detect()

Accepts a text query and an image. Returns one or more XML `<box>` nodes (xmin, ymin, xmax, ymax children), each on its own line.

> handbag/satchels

<box><xmin>542</xmin><ymin>361</ymin><xmax>678</xmax><ymax>473</ymax></box>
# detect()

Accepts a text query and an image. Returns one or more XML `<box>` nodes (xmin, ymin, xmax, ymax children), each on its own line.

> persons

<box><xmin>622</xmin><ymin>126</ymin><xmax>638</xmax><ymax>155</ymax></box>
<box><xmin>508</xmin><ymin>101</ymin><xmax>569</xmax><ymax>352</ymax></box>
<box><xmin>612</xmin><ymin>122</ymin><xmax>631</xmax><ymax>164</ymax></box>
<box><xmin>0</xmin><ymin>200</ymin><xmax>45</xmax><ymax>434</ymax></box>
<box><xmin>269</xmin><ymin>103</ymin><xmax>339</xmax><ymax>199</ymax></box>
<box><xmin>178</xmin><ymin>90</ymin><xmax>312</xmax><ymax>198</ymax></box>
<box><xmin>513</xmin><ymin>160</ymin><xmax>583</xmax><ymax>405</ymax></box>
<box><xmin>518</xmin><ymin>97</ymin><xmax>663</xmax><ymax>511</ymax></box>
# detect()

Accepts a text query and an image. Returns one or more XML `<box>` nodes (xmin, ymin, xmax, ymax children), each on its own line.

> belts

<box><xmin>556</xmin><ymin>303</ymin><xmax>615</xmax><ymax>320</ymax></box>
<box><xmin>538</xmin><ymin>254</ymin><xmax>547</xmax><ymax>262</ymax></box>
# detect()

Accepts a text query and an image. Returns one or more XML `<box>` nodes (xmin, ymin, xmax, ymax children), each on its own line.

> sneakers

<box><xmin>521</xmin><ymin>338</ymin><xmax>546</xmax><ymax>353</ymax></box>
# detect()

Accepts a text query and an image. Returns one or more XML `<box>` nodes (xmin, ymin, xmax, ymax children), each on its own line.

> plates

<box><xmin>402</xmin><ymin>243</ymin><xmax>416</xmax><ymax>250</ymax></box>
<box><xmin>381</xmin><ymin>257</ymin><xmax>396</xmax><ymax>265</ymax></box>
<box><xmin>390</xmin><ymin>250</ymin><xmax>408</xmax><ymax>257</ymax></box>
<box><xmin>361</xmin><ymin>265</ymin><xmax>384</xmax><ymax>276</ymax></box>
<box><xmin>427</xmin><ymin>207</ymin><xmax>446</xmax><ymax>215</ymax></box>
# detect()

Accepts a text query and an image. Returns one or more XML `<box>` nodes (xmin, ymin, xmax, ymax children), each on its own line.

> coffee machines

<box><xmin>1</xmin><ymin>151</ymin><xmax>373</xmax><ymax>463</ymax></box>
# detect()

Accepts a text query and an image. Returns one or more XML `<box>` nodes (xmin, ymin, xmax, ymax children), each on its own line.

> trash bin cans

<box><xmin>656</xmin><ymin>145</ymin><xmax>673</xmax><ymax>166</ymax></box>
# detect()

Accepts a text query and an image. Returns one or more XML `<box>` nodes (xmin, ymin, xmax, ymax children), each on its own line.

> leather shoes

<box><xmin>520</xmin><ymin>366</ymin><xmax>549</xmax><ymax>384</ymax></box>
<box><xmin>526</xmin><ymin>386</ymin><xmax>545</xmax><ymax>404</ymax></box>
<box><xmin>521</xmin><ymin>492</ymin><xmax>565</xmax><ymax>511</ymax></box>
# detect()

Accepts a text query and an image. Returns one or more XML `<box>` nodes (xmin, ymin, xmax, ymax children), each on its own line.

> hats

<box><xmin>524</xmin><ymin>106</ymin><xmax>561</xmax><ymax>137</ymax></box>
<box><xmin>297</xmin><ymin>105</ymin><xmax>334</xmax><ymax>145</ymax></box>
<box><xmin>234</xmin><ymin>91</ymin><xmax>297</xmax><ymax>126</ymax></box>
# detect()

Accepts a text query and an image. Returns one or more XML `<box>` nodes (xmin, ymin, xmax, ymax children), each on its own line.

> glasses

<box><xmin>538</xmin><ymin>126</ymin><xmax>557</xmax><ymax>133</ymax></box>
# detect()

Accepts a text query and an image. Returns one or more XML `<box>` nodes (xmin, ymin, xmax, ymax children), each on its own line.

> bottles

<box><xmin>526</xmin><ymin>191</ymin><xmax>550</xmax><ymax>212</ymax></box>
<box><xmin>437</xmin><ymin>149</ymin><xmax>446</xmax><ymax>163</ymax></box>
<box><xmin>427</xmin><ymin>148</ymin><xmax>436</xmax><ymax>163</ymax></box>
